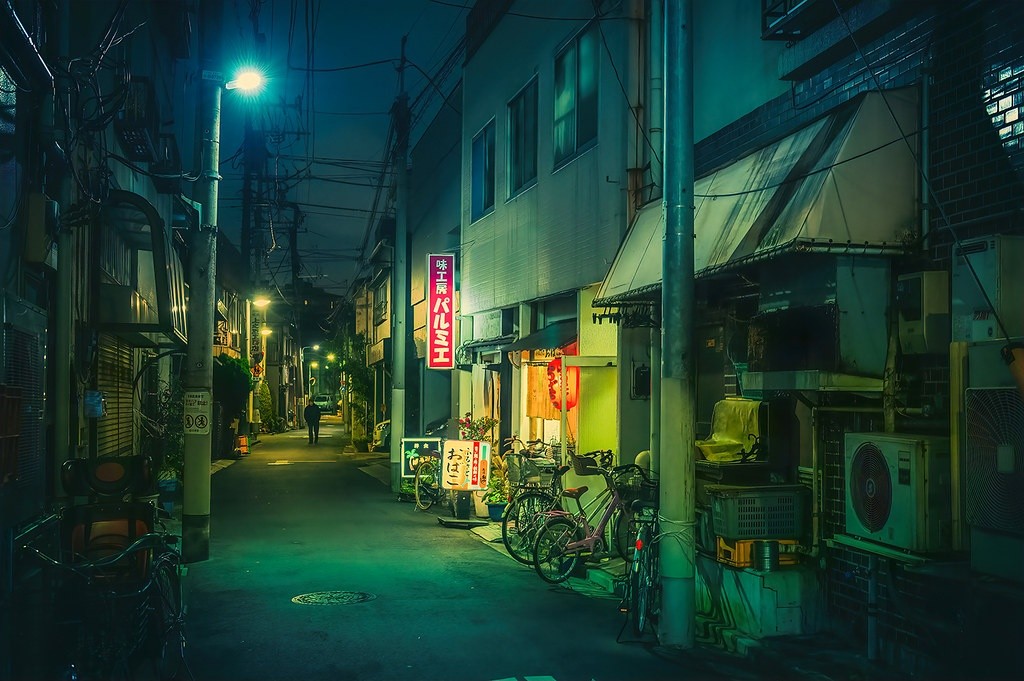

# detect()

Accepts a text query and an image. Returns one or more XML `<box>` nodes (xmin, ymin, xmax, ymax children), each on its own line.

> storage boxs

<box><xmin>705</xmin><ymin>490</ymin><xmax>808</xmax><ymax>540</ymax></box>
<box><xmin>716</xmin><ymin>536</ymin><xmax>800</xmax><ymax>567</ymax></box>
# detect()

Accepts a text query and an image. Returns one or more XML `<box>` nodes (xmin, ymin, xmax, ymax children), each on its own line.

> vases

<box><xmin>474</xmin><ymin>490</ymin><xmax>490</xmax><ymax>517</ymax></box>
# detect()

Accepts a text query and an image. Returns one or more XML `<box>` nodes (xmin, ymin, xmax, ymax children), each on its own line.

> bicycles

<box><xmin>25</xmin><ymin>532</ymin><xmax>196</xmax><ymax>681</ymax></box>
<box><xmin>501</xmin><ymin>435</ymin><xmax>641</xmax><ymax>584</ymax></box>
<box><xmin>413</xmin><ymin>450</ymin><xmax>458</xmax><ymax>518</ymax></box>
<box><xmin>612</xmin><ymin>463</ymin><xmax>662</xmax><ymax>647</ymax></box>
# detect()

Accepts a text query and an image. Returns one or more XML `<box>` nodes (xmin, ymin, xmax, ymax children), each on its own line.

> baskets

<box><xmin>615</xmin><ymin>480</ymin><xmax>660</xmax><ymax>511</ymax></box>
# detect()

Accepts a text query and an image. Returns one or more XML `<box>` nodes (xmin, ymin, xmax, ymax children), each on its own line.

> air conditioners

<box><xmin>843</xmin><ymin>432</ymin><xmax>967</xmax><ymax>555</ymax></box>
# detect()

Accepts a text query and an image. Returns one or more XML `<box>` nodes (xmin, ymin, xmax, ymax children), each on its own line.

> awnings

<box><xmin>501</xmin><ymin>321</ymin><xmax>577</xmax><ymax>352</ymax></box>
<box><xmin>592</xmin><ymin>87</ymin><xmax>921</xmax><ymax>306</ymax></box>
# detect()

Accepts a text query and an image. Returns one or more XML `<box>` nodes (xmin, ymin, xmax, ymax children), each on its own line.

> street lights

<box><xmin>299</xmin><ymin>345</ymin><xmax>319</xmax><ymax>429</ymax></box>
<box><xmin>179</xmin><ymin>71</ymin><xmax>264</xmax><ymax>562</ymax></box>
<box><xmin>309</xmin><ymin>362</ymin><xmax>318</xmax><ymax>400</ymax></box>
<box><xmin>246</xmin><ymin>298</ymin><xmax>272</xmax><ymax>436</ymax></box>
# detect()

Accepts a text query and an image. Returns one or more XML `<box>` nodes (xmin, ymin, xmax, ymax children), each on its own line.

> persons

<box><xmin>304</xmin><ymin>398</ymin><xmax>321</xmax><ymax>445</ymax></box>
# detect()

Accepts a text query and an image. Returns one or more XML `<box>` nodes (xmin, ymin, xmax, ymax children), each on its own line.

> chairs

<box><xmin>61</xmin><ymin>451</ymin><xmax>156</xmax><ymax>574</ymax></box>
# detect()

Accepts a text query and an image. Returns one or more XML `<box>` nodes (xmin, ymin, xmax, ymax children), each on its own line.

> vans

<box><xmin>314</xmin><ymin>395</ymin><xmax>334</xmax><ymax>411</ymax></box>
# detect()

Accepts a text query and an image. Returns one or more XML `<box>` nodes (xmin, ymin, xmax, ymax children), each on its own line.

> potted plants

<box><xmin>483</xmin><ymin>451</ymin><xmax>511</xmax><ymax>520</ymax></box>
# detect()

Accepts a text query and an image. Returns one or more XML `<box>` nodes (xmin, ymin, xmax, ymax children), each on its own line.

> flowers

<box><xmin>454</xmin><ymin>412</ymin><xmax>500</xmax><ymax>447</ymax></box>
